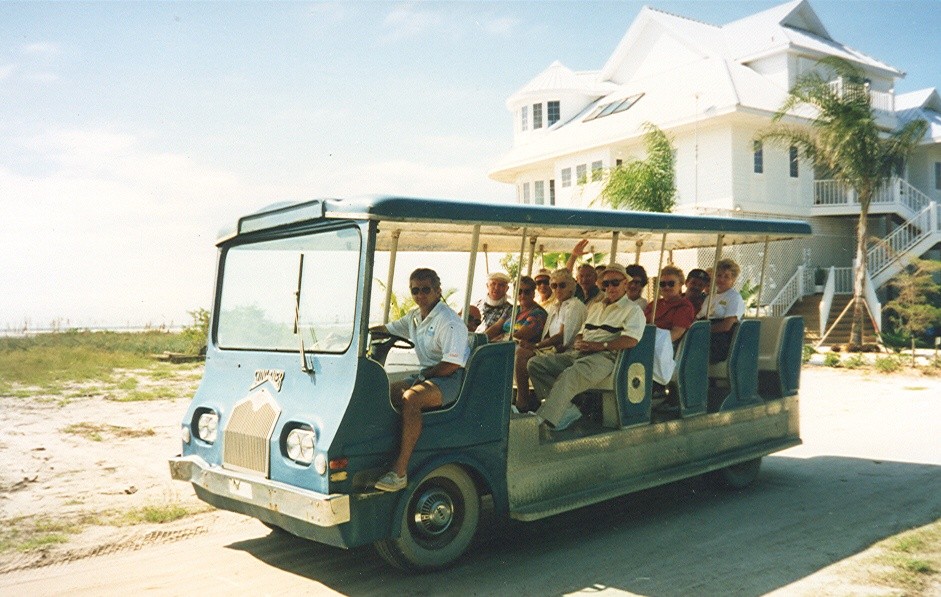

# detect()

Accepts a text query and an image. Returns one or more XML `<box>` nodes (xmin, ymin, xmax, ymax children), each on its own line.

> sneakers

<box><xmin>551</xmin><ymin>404</ymin><xmax>582</xmax><ymax>431</ymax></box>
<box><xmin>376</xmin><ymin>472</ymin><xmax>408</xmax><ymax>492</ymax></box>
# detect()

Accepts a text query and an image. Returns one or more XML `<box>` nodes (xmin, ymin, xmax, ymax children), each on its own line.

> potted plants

<box><xmin>813</xmin><ymin>265</ymin><xmax>825</xmax><ymax>293</ymax></box>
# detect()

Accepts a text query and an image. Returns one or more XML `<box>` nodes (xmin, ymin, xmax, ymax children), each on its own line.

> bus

<box><xmin>167</xmin><ymin>191</ymin><xmax>809</xmax><ymax>574</ymax></box>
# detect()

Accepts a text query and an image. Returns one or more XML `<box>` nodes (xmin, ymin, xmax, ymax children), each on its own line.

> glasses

<box><xmin>660</xmin><ymin>281</ymin><xmax>679</xmax><ymax>287</ymax></box>
<box><xmin>631</xmin><ymin>280</ymin><xmax>643</xmax><ymax>286</ymax></box>
<box><xmin>551</xmin><ymin>282</ymin><xmax>566</xmax><ymax>289</ymax></box>
<box><xmin>604</xmin><ymin>278</ymin><xmax>626</xmax><ymax>287</ymax></box>
<box><xmin>412</xmin><ymin>287</ymin><xmax>431</xmax><ymax>295</ymax></box>
<box><xmin>468</xmin><ymin>315</ymin><xmax>482</xmax><ymax>326</ymax></box>
<box><xmin>519</xmin><ymin>287</ymin><xmax>534</xmax><ymax>296</ymax></box>
<box><xmin>536</xmin><ymin>279</ymin><xmax>549</xmax><ymax>285</ymax></box>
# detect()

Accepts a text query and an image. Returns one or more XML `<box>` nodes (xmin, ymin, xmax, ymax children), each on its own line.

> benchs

<box><xmin>746</xmin><ymin>316</ymin><xmax>802</xmax><ymax>398</ymax></box>
<box><xmin>670</xmin><ymin>320</ymin><xmax>711</xmax><ymax>418</ymax></box>
<box><xmin>512</xmin><ymin>324</ymin><xmax>656</xmax><ymax>429</ymax></box>
<box><xmin>708</xmin><ymin>320</ymin><xmax>763</xmax><ymax>413</ymax></box>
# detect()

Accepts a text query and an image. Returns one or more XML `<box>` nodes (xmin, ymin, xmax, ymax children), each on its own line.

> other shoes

<box><xmin>656</xmin><ymin>398</ymin><xmax>680</xmax><ymax>411</ymax></box>
<box><xmin>515</xmin><ymin>400</ymin><xmax>535</xmax><ymax>412</ymax></box>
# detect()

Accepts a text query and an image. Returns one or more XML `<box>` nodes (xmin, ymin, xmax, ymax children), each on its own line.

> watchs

<box><xmin>418</xmin><ymin>374</ymin><xmax>425</xmax><ymax>381</ymax></box>
<box><xmin>603</xmin><ymin>342</ymin><xmax>608</xmax><ymax>350</ymax></box>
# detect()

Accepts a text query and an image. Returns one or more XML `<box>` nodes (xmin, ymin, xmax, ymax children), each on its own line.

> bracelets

<box><xmin>535</xmin><ymin>342</ymin><xmax>539</xmax><ymax>348</ymax></box>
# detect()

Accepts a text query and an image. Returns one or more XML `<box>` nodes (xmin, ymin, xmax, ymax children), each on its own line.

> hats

<box><xmin>687</xmin><ymin>269</ymin><xmax>709</xmax><ymax>282</ymax></box>
<box><xmin>488</xmin><ymin>272</ymin><xmax>510</xmax><ymax>284</ymax></box>
<box><xmin>595</xmin><ymin>264</ymin><xmax>606</xmax><ymax>269</ymax></box>
<box><xmin>534</xmin><ymin>269</ymin><xmax>551</xmax><ymax>280</ymax></box>
<box><xmin>599</xmin><ymin>263</ymin><xmax>633</xmax><ymax>283</ymax></box>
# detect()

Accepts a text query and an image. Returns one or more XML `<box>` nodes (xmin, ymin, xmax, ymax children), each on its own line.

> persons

<box><xmin>526</xmin><ymin>263</ymin><xmax>646</xmax><ymax>431</ymax></box>
<box><xmin>361</xmin><ymin>268</ymin><xmax>470</xmax><ymax>491</ymax></box>
<box><xmin>510</xmin><ymin>268</ymin><xmax>588</xmax><ymax>417</ymax></box>
<box><xmin>681</xmin><ymin>268</ymin><xmax>710</xmax><ymax>313</ymax></box>
<box><xmin>705</xmin><ymin>267</ymin><xmax>714</xmax><ymax>296</ymax></box>
<box><xmin>469</xmin><ymin>272</ymin><xmax>514</xmax><ymax>343</ymax></box>
<box><xmin>492</xmin><ymin>276</ymin><xmax>548</xmax><ymax>355</ymax></box>
<box><xmin>814</xmin><ymin>265</ymin><xmax>826</xmax><ymax>296</ymax></box>
<box><xmin>456</xmin><ymin>305</ymin><xmax>482</xmax><ymax>332</ymax></box>
<box><xmin>623</xmin><ymin>264</ymin><xmax>649</xmax><ymax>310</ymax></box>
<box><xmin>534</xmin><ymin>239</ymin><xmax>606</xmax><ymax>313</ymax></box>
<box><xmin>645</xmin><ymin>265</ymin><xmax>696</xmax><ymax>397</ymax></box>
<box><xmin>697</xmin><ymin>258</ymin><xmax>747</xmax><ymax>365</ymax></box>
